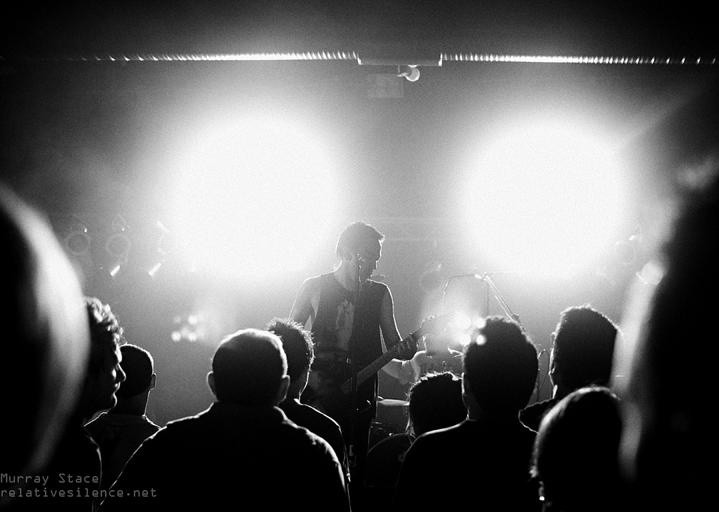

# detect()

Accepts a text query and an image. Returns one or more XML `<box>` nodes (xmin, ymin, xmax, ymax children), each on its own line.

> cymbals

<box><xmin>377</xmin><ymin>398</ymin><xmax>410</xmax><ymax>407</ymax></box>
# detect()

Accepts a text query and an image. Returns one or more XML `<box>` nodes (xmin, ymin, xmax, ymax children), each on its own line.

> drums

<box><xmin>365</xmin><ymin>427</ymin><xmax>416</xmax><ymax>512</ymax></box>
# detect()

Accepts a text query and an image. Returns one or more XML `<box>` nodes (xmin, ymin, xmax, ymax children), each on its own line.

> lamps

<box><xmin>396</xmin><ymin>62</ymin><xmax>421</xmax><ymax>83</ymax></box>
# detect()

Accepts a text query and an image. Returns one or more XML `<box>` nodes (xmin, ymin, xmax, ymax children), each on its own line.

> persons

<box><xmin>0</xmin><ymin>166</ymin><xmax>719</xmax><ymax>511</ymax></box>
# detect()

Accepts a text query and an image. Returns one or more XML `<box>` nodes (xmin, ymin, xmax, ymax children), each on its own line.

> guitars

<box><xmin>300</xmin><ymin>313</ymin><xmax>439</xmax><ymax>420</ymax></box>
